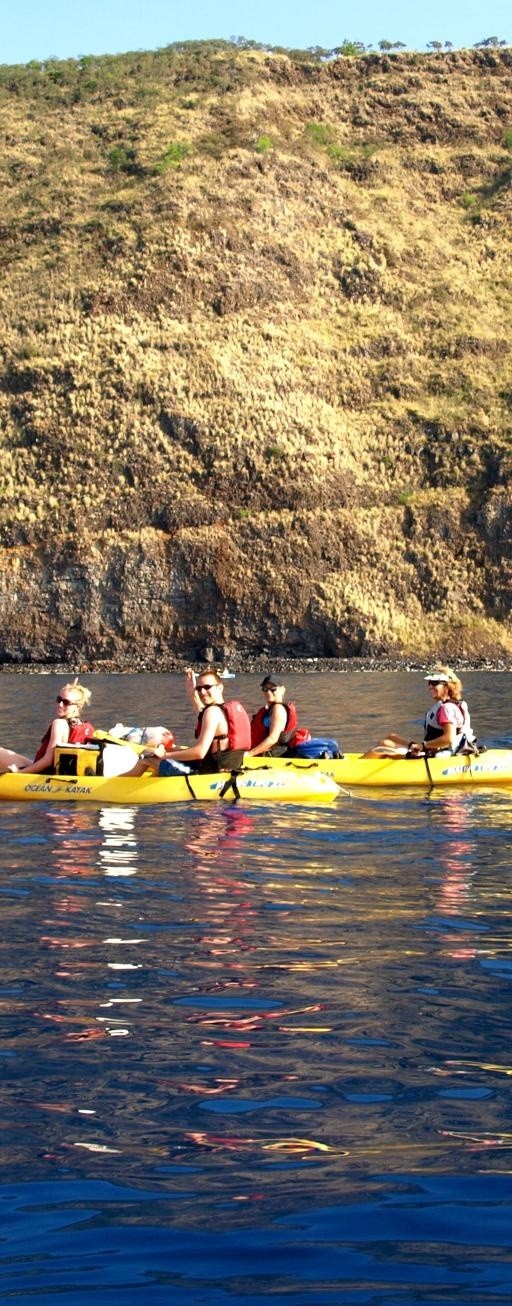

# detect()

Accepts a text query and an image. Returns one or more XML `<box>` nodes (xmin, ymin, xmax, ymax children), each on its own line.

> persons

<box><xmin>116</xmin><ymin>671</ymin><xmax>251</xmax><ymax>776</ymax></box>
<box><xmin>0</xmin><ymin>677</ymin><xmax>92</xmax><ymax>773</ymax></box>
<box><xmin>358</xmin><ymin>666</ymin><xmax>471</xmax><ymax>759</ymax></box>
<box><xmin>244</xmin><ymin>674</ymin><xmax>297</xmax><ymax>756</ymax></box>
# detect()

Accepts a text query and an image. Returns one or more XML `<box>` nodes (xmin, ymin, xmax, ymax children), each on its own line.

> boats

<box><xmin>178</xmin><ymin>746</ymin><xmax>512</xmax><ymax>786</ymax></box>
<box><xmin>0</xmin><ymin>772</ymin><xmax>342</xmax><ymax>805</ymax></box>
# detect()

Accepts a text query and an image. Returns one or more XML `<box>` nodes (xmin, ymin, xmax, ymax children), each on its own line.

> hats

<box><xmin>424</xmin><ymin>671</ymin><xmax>452</xmax><ymax>682</ymax></box>
<box><xmin>260</xmin><ymin>675</ymin><xmax>283</xmax><ymax>686</ymax></box>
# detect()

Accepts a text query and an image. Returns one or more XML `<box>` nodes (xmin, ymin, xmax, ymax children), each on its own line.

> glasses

<box><xmin>195</xmin><ymin>685</ymin><xmax>211</xmax><ymax>691</ymax></box>
<box><xmin>57</xmin><ymin>696</ymin><xmax>70</xmax><ymax>705</ymax></box>
<box><xmin>262</xmin><ymin>687</ymin><xmax>277</xmax><ymax>692</ymax></box>
<box><xmin>428</xmin><ymin>681</ymin><xmax>440</xmax><ymax>685</ymax></box>
